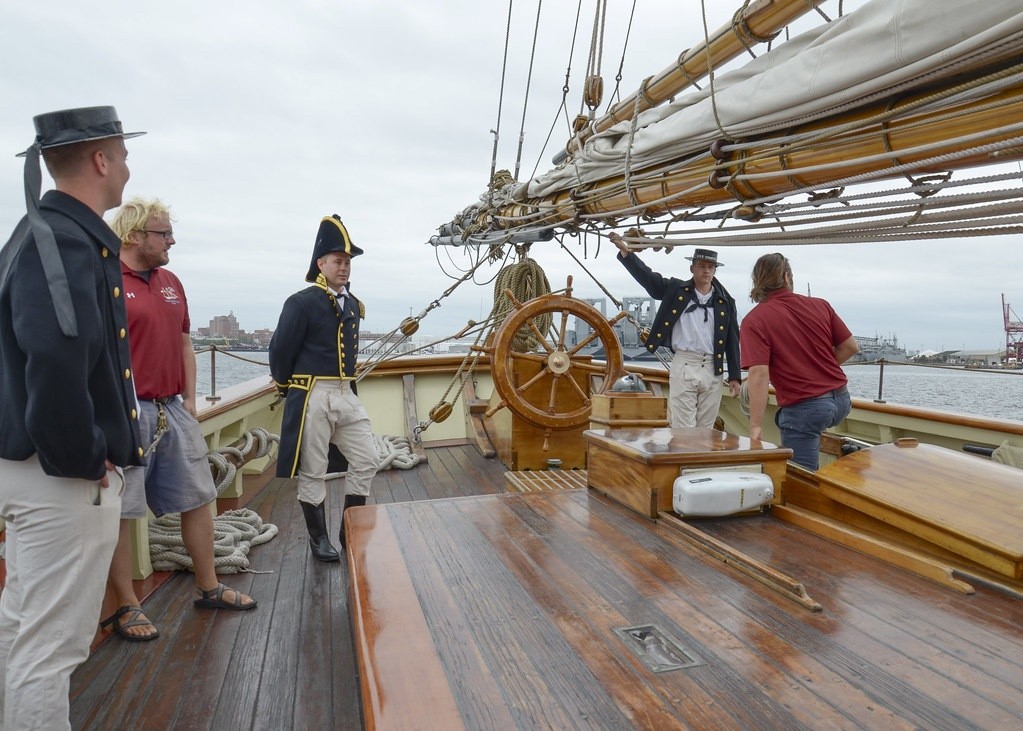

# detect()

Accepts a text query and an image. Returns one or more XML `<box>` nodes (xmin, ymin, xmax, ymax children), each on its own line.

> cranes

<box><xmin>1001</xmin><ymin>292</ymin><xmax>1023</xmax><ymax>372</ymax></box>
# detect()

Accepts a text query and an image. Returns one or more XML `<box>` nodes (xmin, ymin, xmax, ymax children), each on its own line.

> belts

<box><xmin>139</xmin><ymin>394</ymin><xmax>175</xmax><ymax>404</ymax></box>
<box><xmin>811</xmin><ymin>384</ymin><xmax>847</xmax><ymax>398</ymax></box>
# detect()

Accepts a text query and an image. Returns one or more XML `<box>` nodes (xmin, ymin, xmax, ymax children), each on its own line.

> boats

<box><xmin>192</xmin><ymin>335</ymin><xmax>268</xmax><ymax>351</ymax></box>
<box><xmin>860</xmin><ymin>330</ymin><xmax>907</xmax><ymax>364</ymax></box>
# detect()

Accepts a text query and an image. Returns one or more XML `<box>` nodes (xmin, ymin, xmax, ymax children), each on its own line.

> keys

<box><xmin>155</xmin><ymin>402</ymin><xmax>169</xmax><ymax>434</ymax></box>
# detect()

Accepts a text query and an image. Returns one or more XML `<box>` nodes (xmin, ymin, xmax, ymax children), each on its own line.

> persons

<box><xmin>109</xmin><ymin>198</ymin><xmax>259</xmax><ymax>640</ymax></box>
<box><xmin>269</xmin><ymin>214</ymin><xmax>380</xmax><ymax>562</ymax></box>
<box><xmin>0</xmin><ymin>105</ymin><xmax>145</xmax><ymax>731</ymax></box>
<box><xmin>609</xmin><ymin>232</ymin><xmax>741</xmax><ymax>429</ymax></box>
<box><xmin>739</xmin><ymin>253</ymin><xmax>859</xmax><ymax>471</ymax></box>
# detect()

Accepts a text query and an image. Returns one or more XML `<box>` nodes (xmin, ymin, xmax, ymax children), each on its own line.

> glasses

<box><xmin>132</xmin><ymin>229</ymin><xmax>174</xmax><ymax>239</ymax></box>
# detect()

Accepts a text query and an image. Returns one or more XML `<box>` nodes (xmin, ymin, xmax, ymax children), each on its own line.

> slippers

<box><xmin>113</xmin><ymin>604</ymin><xmax>160</xmax><ymax>641</ymax></box>
<box><xmin>194</xmin><ymin>581</ymin><xmax>257</xmax><ymax>610</ymax></box>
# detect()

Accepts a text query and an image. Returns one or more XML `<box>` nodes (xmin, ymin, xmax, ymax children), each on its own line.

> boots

<box><xmin>299</xmin><ymin>497</ymin><xmax>339</xmax><ymax>562</ymax></box>
<box><xmin>338</xmin><ymin>494</ymin><xmax>366</xmax><ymax>548</ymax></box>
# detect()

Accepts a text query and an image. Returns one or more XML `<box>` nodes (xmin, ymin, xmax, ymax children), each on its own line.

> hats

<box><xmin>305</xmin><ymin>214</ymin><xmax>364</xmax><ymax>284</ymax></box>
<box><xmin>15</xmin><ymin>106</ymin><xmax>147</xmax><ymax>156</ymax></box>
<box><xmin>684</xmin><ymin>249</ymin><xmax>725</xmax><ymax>266</ymax></box>
